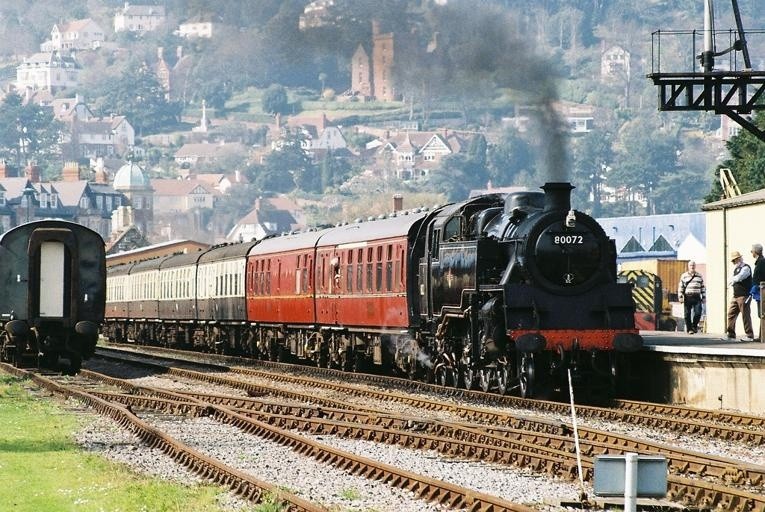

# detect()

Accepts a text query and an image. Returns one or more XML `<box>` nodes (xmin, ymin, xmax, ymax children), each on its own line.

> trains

<box><xmin>97</xmin><ymin>178</ymin><xmax>649</xmax><ymax>405</ymax></box>
<box><xmin>0</xmin><ymin>214</ymin><xmax>114</xmax><ymax>379</ymax></box>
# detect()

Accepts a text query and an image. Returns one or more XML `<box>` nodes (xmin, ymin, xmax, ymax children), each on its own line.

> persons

<box><xmin>720</xmin><ymin>251</ymin><xmax>754</xmax><ymax>342</ymax></box>
<box><xmin>678</xmin><ymin>261</ymin><xmax>706</xmax><ymax>334</ymax></box>
<box><xmin>750</xmin><ymin>245</ymin><xmax>765</xmax><ymax>342</ymax></box>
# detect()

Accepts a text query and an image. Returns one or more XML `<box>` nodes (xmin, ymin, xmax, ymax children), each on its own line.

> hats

<box><xmin>729</xmin><ymin>251</ymin><xmax>743</xmax><ymax>263</ymax></box>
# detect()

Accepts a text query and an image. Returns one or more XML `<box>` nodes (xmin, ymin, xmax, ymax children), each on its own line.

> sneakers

<box><xmin>740</xmin><ymin>332</ymin><xmax>754</xmax><ymax>343</ymax></box>
<box><xmin>720</xmin><ymin>334</ymin><xmax>736</xmax><ymax>342</ymax></box>
<box><xmin>688</xmin><ymin>326</ymin><xmax>698</xmax><ymax>335</ymax></box>
